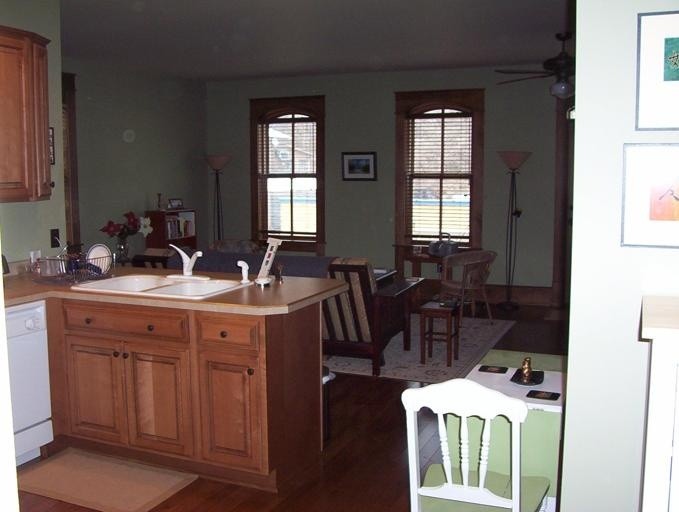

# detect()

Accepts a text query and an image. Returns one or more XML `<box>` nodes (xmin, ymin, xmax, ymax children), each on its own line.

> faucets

<box><xmin>236</xmin><ymin>260</ymin><xmax>250</xmax><ymax>285</ymax></box>
<box><xmin>168</xmin><ymin>244</ymin><xmax>203</xmax><ymax>277</ymax></box>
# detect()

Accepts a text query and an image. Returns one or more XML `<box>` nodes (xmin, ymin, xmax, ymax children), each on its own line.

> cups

<box><xmin>40</xmin><ymin>258</ymin><xmax>57</xmax><ymax>276</ymax></box>
<box><xmin>413</xmin><ymin>245</ymin><xmax>422</xmax><ymax>254</ymax></box>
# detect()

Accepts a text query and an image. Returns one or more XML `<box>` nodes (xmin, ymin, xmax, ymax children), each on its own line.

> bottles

<box><xmin>522</xmin><ymin>356</ymin><xmax>532</xmax><ymax>381</ymax></box>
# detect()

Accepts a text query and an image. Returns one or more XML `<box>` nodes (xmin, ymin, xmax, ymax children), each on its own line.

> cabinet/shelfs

<box><xmin>637</xmin><ymin>294</ymin><xmax>679</xmax><ymax>512</ymax></box>
<box><xmin>53</xmin><ymin>299</ymin><xmax>199</xmax><ymax>477</ymax></box>
<box><xmin>447</xmin><ymin>349</ymin><xmax>567</xmax><ymax>512</ymax></box>
<box><xmin>1</xmin><ymin>26</ymin><xmax>52</xmax><ymax>206</ymax></box>
<box><xmin>195</xmin><ymin>299</ymin><xmax>325</xmax><ymax>495</ymax></box>
<box><xmin>143</xmin><ymin>208</ymin><xmax>198</xmax><ymax>252</ymax></box>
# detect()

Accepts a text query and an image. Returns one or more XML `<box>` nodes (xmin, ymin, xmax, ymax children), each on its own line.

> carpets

<box><xmin>322</xmin><ymin>314</ymin><xmax>515</xmax><ymax>385</ymax></box>
<box><xmin>19</xmin><ymin>448</ymin><xmax>197</xmax><ymax>512</ymax></box>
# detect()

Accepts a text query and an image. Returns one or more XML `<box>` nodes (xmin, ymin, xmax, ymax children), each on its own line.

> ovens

<box><xmin>5</xmin><ymin>298</ymin><xmax>55</xmax><ymax>468</ymax></box>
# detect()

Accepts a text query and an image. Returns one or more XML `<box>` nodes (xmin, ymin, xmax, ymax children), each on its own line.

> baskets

<box><xmin>37</xmin><ymin>252</ymin><xmax>116</xmax><ymax>284</ymax></box>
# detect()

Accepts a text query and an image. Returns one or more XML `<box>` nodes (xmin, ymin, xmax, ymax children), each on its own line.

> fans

<box><xmin>493</xmin><ymin>33</ymin><xmax>575</xmax><ymax>104</ymax></box>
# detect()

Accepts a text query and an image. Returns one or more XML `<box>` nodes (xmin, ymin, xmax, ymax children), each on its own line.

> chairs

<box><xmin>398</xmin><ymin>378</ymin><xmax>551</xmax><ymax>512</ymax></box>
<box><xmin>440</xmin><ymin>251</ymin><xmax>497</xmax><ymax>327</ymax></box>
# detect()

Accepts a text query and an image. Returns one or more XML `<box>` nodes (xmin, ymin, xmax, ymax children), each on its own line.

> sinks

<box><xmin>70</xmin><ymin>272</ymin><xmax>176</xmax><ymax>297</ymax></box>
<box><xmin>146</xmin><ymin>280</ymin><xmax>241</xmax><ymax>300</ymax></box>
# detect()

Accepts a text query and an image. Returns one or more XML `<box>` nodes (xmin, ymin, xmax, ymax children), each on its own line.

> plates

<box><xmin>86</xmin><ymin>243</ymin><xmax>112</xmax><ymax>274</ymax></box>
<box><xmin>509</xmin><ymin>367</ymin><xmax>544</xmax><ymax>386</ymax></box>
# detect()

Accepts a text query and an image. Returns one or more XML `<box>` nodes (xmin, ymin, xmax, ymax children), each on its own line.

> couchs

<box><xmin>133</xmin><ymin>248</ymin><xmax>425</xmax><ymax>378</ymax></box>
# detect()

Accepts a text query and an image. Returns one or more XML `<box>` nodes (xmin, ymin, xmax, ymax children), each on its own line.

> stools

<box><xmin>420</xmin><ymin>301</ymin><xmax>461</xmax><ymax>367</ymax></box>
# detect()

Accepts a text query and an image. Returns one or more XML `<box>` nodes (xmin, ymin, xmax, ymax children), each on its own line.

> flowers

<box><xmin>99</xmin><ymin>211</ymin><xmax>155</xmax><ymax>241</ymax></box>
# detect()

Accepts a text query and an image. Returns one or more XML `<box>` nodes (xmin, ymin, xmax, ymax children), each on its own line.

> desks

<box><xmin>391</xmin><ymin>243</ymin><xmax>453</xmax><ymax>303</ymax></box>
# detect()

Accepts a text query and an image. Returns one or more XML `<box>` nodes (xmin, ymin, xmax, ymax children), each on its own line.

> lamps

<box><xmin>548</xmin><ymin>73</ymin><xmax>575</xmax><ymax>104</ymax></box>
<box><xmin>208</xmin><ymin>150</ymin><xmax>235</xmax><ymax>240</ymax></box>
<box><xmin>500</xmin><ymin>151</ymin><xmax>530</xmax><ymax>311</ymax></box>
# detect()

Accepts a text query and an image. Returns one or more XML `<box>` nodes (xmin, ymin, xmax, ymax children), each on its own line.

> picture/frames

<box><xmin>620</xmin><ymin>140</ymin><xmax>679</xmax><ymax>248</ymax></box>
<box><xmin>169</xmin><ymin>198</ymin><xmax>183</xmax><ymax>208</ymax></box>
<box><xmin>340</xmin><ymin>151</ymin><xmax>377</xmax><ymax>183</ymax></box>
<box><xmin>634</xmin><ymin>10</ymin><xmax>679</xmax><ymax>131</ymax></box>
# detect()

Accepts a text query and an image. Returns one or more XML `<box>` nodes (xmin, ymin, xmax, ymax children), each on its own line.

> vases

<box><xmin>115</xmin><ymin>240</ymin><xmax>129</xmax><ymax>260</ymax></box>
